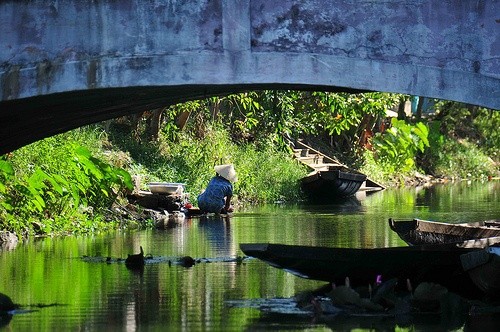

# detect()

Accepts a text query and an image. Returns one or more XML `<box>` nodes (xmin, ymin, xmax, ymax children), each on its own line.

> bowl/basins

<box><xmin>149</xmin><ymin>183</ymin><xmax>187</xmax><ymax>193</ymax></box>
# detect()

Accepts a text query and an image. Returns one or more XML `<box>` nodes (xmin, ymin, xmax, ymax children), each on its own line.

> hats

<box><xmin>214</xmin><ymin>164</ymin><xmax>237</xmax><ymax>182</ymax></box>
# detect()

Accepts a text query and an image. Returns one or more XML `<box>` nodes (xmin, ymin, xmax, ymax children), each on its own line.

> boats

<box><xmin>236</xmin><ymin>240</ymin><xmax>500</xmax><ymax>310</ymax></box>
<box><xmin>385</xmin><ymin>211</ymin><xmax>500</xmax><ymax>247</ymax></box>
<box><xmin>295</xmin><ymin>168</ymin><xmax>368</xmax><ymax>205</ymax></box>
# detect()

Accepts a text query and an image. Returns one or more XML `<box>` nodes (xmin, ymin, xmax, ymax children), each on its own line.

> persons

<box><xmin>196</xmin><ymin>163</ymin><xmax>239</xmax><ymax>218</ymax></box>
